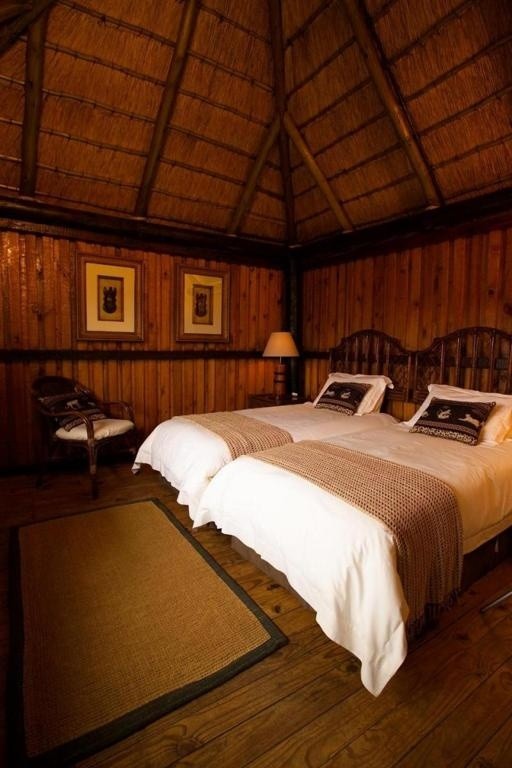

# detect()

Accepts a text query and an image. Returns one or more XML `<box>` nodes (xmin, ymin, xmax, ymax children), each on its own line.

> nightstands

<box><xmin>249</xmin><ymin>394</ymin><xmax>306</xmax><ymax>407</ymax></box>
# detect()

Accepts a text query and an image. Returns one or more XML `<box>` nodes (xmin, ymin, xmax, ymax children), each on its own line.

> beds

<box><xmin>211</xmin><ymin>326</ymin><xmax>512</xmax><ymax>642</ymax></box>
<box><xmin>152</xmin><ymin>328</ymin><xmax>410</xmax><ymax>508</ymax></box>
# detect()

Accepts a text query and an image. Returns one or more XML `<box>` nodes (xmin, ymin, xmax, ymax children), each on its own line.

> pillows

<box><xmin>402</xmin><ymin>384</ymin><xmax>511</xmax><ymax>446</ymax></box>
<box><xmin>313</xmin><ymin>371</ymin><xmax>393</xmax><ymax>416</ymax></box>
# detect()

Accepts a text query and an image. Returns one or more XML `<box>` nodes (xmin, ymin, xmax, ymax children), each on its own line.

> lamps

<box><xmin>263</xmin><ymin>331</ymin><xmax>300</xmax><ymax>400</ymax></box>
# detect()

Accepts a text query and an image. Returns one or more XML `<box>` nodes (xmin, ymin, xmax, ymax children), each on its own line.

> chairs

<box><xmin>31</xmin><ymin>373</ymin><xmax>140</xmax><ymax>500</ymax></box>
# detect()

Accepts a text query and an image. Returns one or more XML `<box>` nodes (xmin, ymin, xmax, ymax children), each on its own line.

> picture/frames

<box><xmin>173</xmin><ymin>264</ymin><xmax>233</xmax><ymax>344</ymax></box>
<box><xmin>75</xmin><ymin>253</ymin><xmax>144</xmax><ymax>343</ymax></box>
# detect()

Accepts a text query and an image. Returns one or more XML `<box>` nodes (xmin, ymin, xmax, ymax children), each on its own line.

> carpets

<box><xmin>7</xmin><ymin>496</ymin><xmax>290</xmax><ymax>767</ymax></box>
